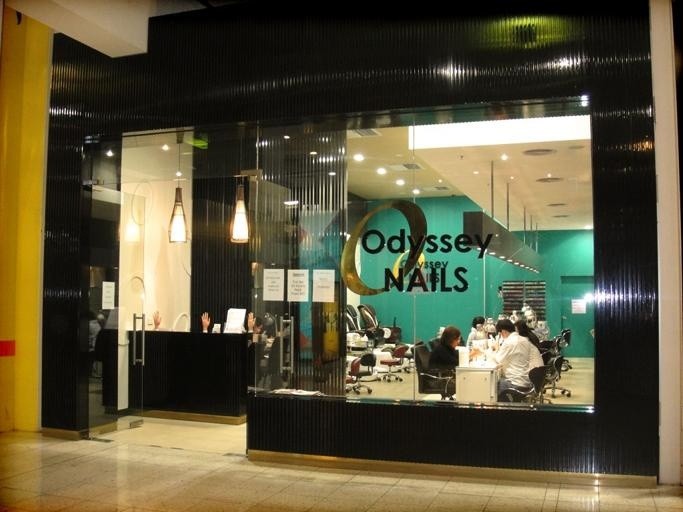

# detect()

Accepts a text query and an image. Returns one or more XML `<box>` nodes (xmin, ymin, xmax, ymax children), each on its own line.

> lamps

<box><xmin>167</xmin><ymin>123</ymin><xmax>248</xmax><ymax>244</ymax></box>
<box><xmin>462</xmin><ymin>159</ymin><xmax>545</xmax><ymax>274</ymax></box>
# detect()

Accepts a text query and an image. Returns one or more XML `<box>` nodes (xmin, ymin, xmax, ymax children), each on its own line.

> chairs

<box><xmin>344</xmin><ymin>303</ymin><xmax>572</xmax><ymax>403</ymax></box>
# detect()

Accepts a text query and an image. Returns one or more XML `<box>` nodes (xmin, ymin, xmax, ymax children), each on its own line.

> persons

<box><xmin>465</xmin><ymin>315</ymin><xmax>487</xmax><ymax>350</ymax></box>
<box><xmin>153</xmin><ymin>311</ymin><xmax>161</xmax><ymax>331</ymax></box>
<box><xmin>201</xmin><ymin>312</ymin><xmax>210</xmax><ymax>333</ymax></box>
<box><xmin>513</xmin><ymin>319</ymin><xmax>540</xmax><ymax>349</ymax></box>
<box><xmin>87</xmin><ymin>310</ymin><xmax>102</xmax><ymax>379</ymax></box>
<box><xmin>247</xmin><ymin>311</ymin><xmax>256</xmax><ymax>333</ymax></box>
<box><xmin>425</xmin><ymin>326</ymin><xmax>480</xmax><ymax>387</ymax></box>
<box><xmin>486</xmin><ymin>318</ymin><xmax>544</xmax><ymax>402</ymax></box>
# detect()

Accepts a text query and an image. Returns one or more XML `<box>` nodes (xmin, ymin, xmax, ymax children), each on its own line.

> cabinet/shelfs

<box><xmin>128</xmin><ymin>328</ymin><xmax>255</xmax><ymax>425</ymax></box>
